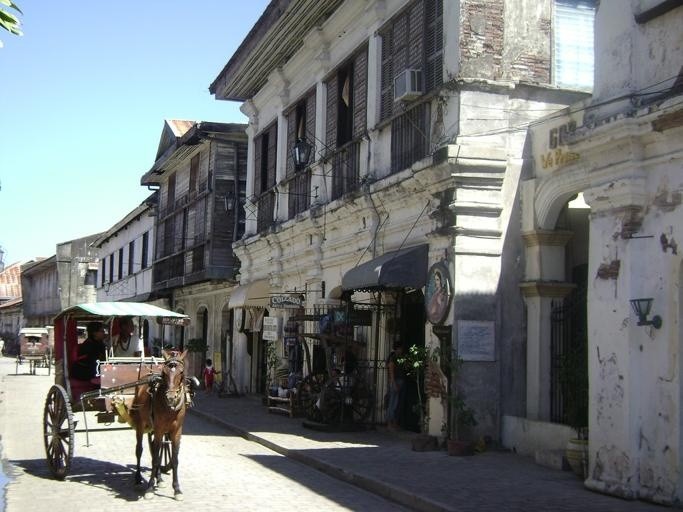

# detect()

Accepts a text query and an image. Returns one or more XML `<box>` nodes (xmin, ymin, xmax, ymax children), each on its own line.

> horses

<box><xmin>129</xmin><ymin>348</ymin><xmax>190</xmax><ymax>500</ymax></box>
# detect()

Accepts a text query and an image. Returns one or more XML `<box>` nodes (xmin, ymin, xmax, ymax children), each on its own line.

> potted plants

<box><xmin>561</xmin><ymin>325</ymin><xmax>589</xmax><ymax>480</ymax></box>
<box><xmin>433</xmin><ymin>344</ymin><xmax>482</xmax><ymax>457</ymax></box>
<box><xmin>397</xmin><ymin>336</ymin><xmax>442</xmax><ymax>455</ymax></box>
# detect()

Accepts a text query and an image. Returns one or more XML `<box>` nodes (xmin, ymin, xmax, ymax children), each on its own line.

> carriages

<box><xmin>14</xmin><ymin>328</ymin><xmax>50</xmax><ymax>375</ymax></box>
<box><xmin>40</xmin><ymin>302</ymin><xmax>200</xmax><ymax>501</ymax></box>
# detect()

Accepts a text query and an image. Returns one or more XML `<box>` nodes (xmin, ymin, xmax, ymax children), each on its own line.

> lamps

<box><xmin>283</xmin><ymin>127</ymin><xmax>365</xmax><ymax>184</ymax></box>
<box><xmin>627</xmin><ymin>298</ymin><xmax>662</xmax><ymax>329</ymax></box>
<box><xmin>100</xmin><ymin>279</ymin><xmax>133</xmax><ymax>295</ymax></box>
<box><xmin>221</xmin><ymin>190</ymin><xmax>262</xmax><ymax>220</ymax></box>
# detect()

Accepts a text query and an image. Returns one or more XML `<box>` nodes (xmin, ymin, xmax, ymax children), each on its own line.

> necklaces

<box><xmin>119</xmin><ymin>335</ymin><xmax>131</xmax><ymax>351</ymax></box>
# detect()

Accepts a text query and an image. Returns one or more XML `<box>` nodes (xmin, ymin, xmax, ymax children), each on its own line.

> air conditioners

<box><xmin>391</xmin><ymin>67</ymin><xmax>424</xmax><ymax>103</ymax></box>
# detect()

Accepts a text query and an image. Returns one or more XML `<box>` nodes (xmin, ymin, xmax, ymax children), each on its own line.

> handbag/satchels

<box><xmin>110</xmin><ymin>316</ymin><xmax>134</xmax><ymax>335</ymax></box>
<box><xmin>72</xmin><ymin>355</ymin><xmax>98</xmax><ymax>382</ymax></box>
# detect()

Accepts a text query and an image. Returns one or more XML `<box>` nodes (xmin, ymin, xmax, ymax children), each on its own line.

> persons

<box><xmin>202</xmin><ymin>358</ymin><xmax>220</xmax><ymax>394</ymax></box>
<box><xmin>77</xmin><ymin>321</ymin><xmax>109</xmax><ymax>385</ymax></box>
<box><xmin>313</xmin><ymin>362</ymin><xmax>353</xmax><ymax>421</ymax></box>
<box><xmin>109</xmin><ymin>317</ymin><xmax>144</xmax><ymax>357</ymax></box>
<box><xmin>427</xmin><ymin>271</ymin><xmax>448</xmax><ymax>322</ymax></box>
<box><xmin>385</xmin><ymin>340</ymin><xmax>407</xmax><ymax>430</ymax></box>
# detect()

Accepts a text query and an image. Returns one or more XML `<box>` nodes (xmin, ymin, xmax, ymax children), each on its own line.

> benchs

<box><xmin>265</xmin><ymin>372</ymin><xmax>311</xmax><ymax>418</ymax></box>
